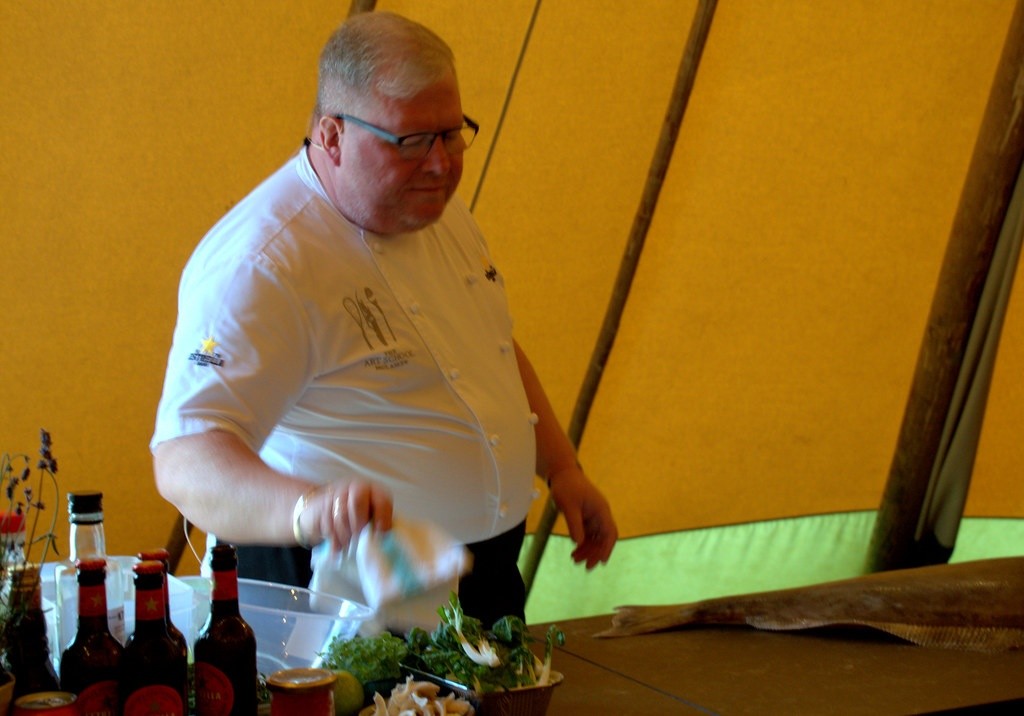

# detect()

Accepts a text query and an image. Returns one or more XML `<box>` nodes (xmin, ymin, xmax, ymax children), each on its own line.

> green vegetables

<box><xmin>316</xmin><ymin>590</ymin><xmax>569</xmax><ymax>704</ymax></box>
<box><xmin>186</xmin><ymin>658</ymin><xmax>271</xmax><ymax>713</ymax></box>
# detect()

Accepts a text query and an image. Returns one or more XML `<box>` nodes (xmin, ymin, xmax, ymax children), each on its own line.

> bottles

<box><xmin>0</xmin><ymin>489</ymin><xmax>258</xmax><ymax>716</ymax></box>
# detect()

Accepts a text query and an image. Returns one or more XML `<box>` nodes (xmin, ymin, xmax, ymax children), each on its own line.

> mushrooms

<box><xmin>368</xmin><ymin>675</ymin><xmax>474</xmax><ymax>716</ymax></box>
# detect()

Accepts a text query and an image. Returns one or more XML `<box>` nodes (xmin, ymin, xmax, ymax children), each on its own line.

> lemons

<box><xmin>327</xmin><ymin>668</ymin><xmax>364</xmax><ymax>716</ymax></box>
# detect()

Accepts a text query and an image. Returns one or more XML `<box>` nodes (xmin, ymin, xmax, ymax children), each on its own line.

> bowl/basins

<box><xmin>178</xmin><ymin>574</ymin><xmax>563</xmax><ymax>716</ymax></box>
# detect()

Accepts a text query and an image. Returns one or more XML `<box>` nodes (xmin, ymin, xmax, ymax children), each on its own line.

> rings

<box><xmin>334</xmin><ymin>499</ymin><xmax>345</xmax><ymax>516</ymax></box>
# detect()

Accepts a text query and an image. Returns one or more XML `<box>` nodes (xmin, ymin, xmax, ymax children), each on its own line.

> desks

<box><xmin>532</xmin><ymin>557</ymin><xmax>1024</xmax><ymax>716</ymax></box>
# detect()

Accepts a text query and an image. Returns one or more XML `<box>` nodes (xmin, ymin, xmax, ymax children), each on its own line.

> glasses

<box><xmin>337</xmin><ymin>114</ymin><xmax>479</xmax><ymax>160</ymax></box>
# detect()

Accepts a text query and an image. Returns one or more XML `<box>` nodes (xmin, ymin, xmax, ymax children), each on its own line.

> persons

<box><xmin>150</xmin><ymin>13</ymin><xmax>617</xmax><ymax>637</ymax></box>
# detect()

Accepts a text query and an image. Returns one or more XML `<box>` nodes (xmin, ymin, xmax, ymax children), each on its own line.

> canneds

<box><xmin>13</xmin><ymin>690</ymin><xmax>80</xmax><ymax>716</ymax></box>
<box><xmin>265</xmin><ymin>668</ymin><xmax>338</xmax><ymax>716</ymax></box>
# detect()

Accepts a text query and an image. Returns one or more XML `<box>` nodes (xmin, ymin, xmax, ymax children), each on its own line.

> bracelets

<box><xmin>293</xmin><ymin>485</ymin><xmax>316</xmax><ymax>549</ymax></box>
<box><xmin>548</xmin><ymin>461</ymin><xmax>583</xmax><ymax>488</ymax></box>
